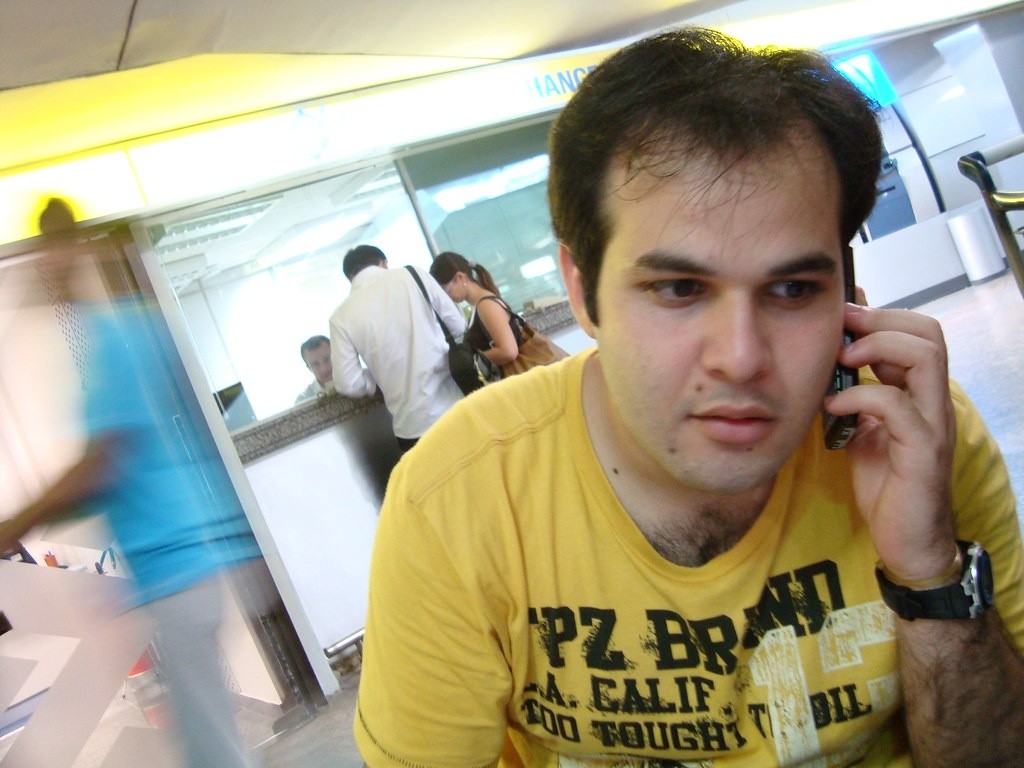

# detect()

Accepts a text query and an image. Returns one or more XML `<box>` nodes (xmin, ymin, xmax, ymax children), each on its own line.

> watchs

<box><xmin>875</xmin><ymin>540</ymin><xmax>994</xmax><ymax>622</ymax></box>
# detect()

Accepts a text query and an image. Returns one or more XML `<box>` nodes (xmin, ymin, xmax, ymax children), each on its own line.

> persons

<box><xmin>353</xmin><ymin>26</ymin><xmax>1024</xmax><ymax>768</ymax></box>
<box><xmin>0</xmin><ymin>197</ymin><xmax>251</xmax><ymax>767</ymax></box>
<box><xmin>430</xmin><ymin>252</ymin><xmax>523</xmax><ymax>371</ymax></box>
<box><xmin>295</xmin><ymin>336</ymin><xmax>333</xmax><ymax>404</ymax></box>
<box><xmin>330</xmin><ymin>245</ymin><xmax>466</xmax><ymax>453</ymax></box>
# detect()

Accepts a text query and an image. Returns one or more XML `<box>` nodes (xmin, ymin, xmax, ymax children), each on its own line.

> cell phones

<box><xmin>823</xmin><ymin>247</ymin><xmax>859</xmax><ymax>450</ymax></box>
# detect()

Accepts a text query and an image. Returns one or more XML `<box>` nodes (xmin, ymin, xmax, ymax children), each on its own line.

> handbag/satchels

<box><xmin>476</xmin><ymin>297</ymin><xmax>571</xmax><ymax>378</ymax></box>
<box><xmin>447</xmin><ymin>344</ymin><xmax>505</xmax><ymax>397</ymax></box>
